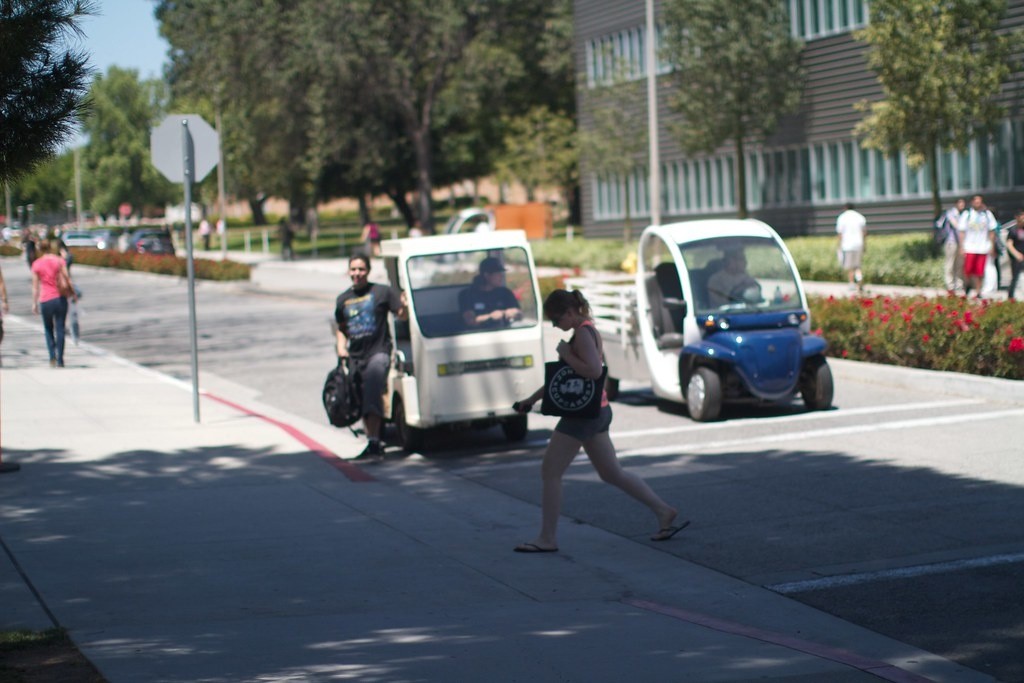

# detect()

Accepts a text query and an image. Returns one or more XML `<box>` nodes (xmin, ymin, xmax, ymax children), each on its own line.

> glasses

<box><xmin>552</xmin><ymin>309</ymin><xmax>568</xmax><ymax>327</ymax></box>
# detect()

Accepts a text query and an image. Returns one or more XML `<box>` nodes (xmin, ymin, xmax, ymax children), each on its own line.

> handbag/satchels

<box><xmin>70</xmin><ymin>302</ymin><xmax>80</xmax><ymax>346</ymax></box>
<box><xmin>540</xmin><ymin>363</ymin><xmax>608</xmax><ymax>419</ymax></box>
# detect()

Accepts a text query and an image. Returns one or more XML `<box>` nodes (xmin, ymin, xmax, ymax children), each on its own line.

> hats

<box><xmin>480</xmin><ymin>257</ymin><xmax>508</xmax><ymax>273</ymax></box>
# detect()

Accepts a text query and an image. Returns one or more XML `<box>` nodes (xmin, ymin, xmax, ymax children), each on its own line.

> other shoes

<box><xmin>50</xmin><ymin>357</ymin><xmax>65</xmax><ymax>370</ymax></box>
<box><xmin>346</xmin><ymin>443</ymin><xmax>385</xmax><ymax>464</ymax></box>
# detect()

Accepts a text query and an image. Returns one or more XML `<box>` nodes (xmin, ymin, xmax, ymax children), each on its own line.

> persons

<box><xmin>407</xmin><ymin>217</ymin><xmax>424</xmax><ymax>239</ymax></box>
<box><xmin>456</xmin><ymin>257</ymin><xmax>524</xmax><ymax>331</ymax></box>
<box><xmin>835</xmin><ymin>201</ymin><xmax>869</xmax><ymax>295</ymax></box>
<box><xmin>475</xmin><ymin>213</ymin><xmax>493</xmax><ymax>231</ymax></box>
<box><xmin>0</xmin><ymin>225</ymin><xmax>80</xmax><ymax>369</ymax></box>
<box><xmin>217</xmin><ymin>216</ymin><xmax>227</xmax><ymax>249</ymax></box>
<box><xmin>513</xmin><ymin>288</ymin><xmax>689</xmax><ymax>554</ymax></box>
<box><xmin>330</xmin><ymin>251</ymin><xmax>411</xmax><ymax>466</ymax></box>
<box><xmin>361</xmin><ymin>216</ymin><xmax>386</xmax><ymax>259</ymax></box>
<box><xmin>943</xmin><ymin>195</ymin><xmax>1024</xmax><ymax>299</ymax></box>
<box><xmin>275</xmin><ymin>220</ymin><xmax>296</xmax><ymax>261</ymax></box>
<box><xmin>708</xmin><ymin>240</ymin><xmax>763</xmax><ymax>306</ymax></box>
<box><xmin>199</xmin><ymin>215</ymin><xmax>212</xmax><ymax>252</ymax></box>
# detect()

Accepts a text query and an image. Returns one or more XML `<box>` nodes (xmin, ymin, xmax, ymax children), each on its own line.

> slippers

<box><xmin>651</xmin><ymin>520</ymin><xmax>692</xmax><ymax>541</ymax></box>
<box><xmin>513</xmin><ymin>541</ymin><xmax>560</xmax><ymax>554</ymax></box>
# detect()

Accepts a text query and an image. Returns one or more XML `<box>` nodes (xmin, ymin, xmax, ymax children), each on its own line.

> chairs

<box><xmin>701</xmin><ymin>259</ymin><xmax>728</xmax><ymax>309</ymax></box>
<box><xmin>654</xmin><ymin>262</ymin><xmax>692</xmax><ymax>332</ymax></box>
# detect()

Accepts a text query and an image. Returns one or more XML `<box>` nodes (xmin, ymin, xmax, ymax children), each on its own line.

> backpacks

<box><xmin>322</xmin><ymin>357</ymin><xmax>366</xmax><ymax>429</ymax></box>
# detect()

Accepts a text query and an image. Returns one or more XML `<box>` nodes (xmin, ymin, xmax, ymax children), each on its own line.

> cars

<box><xmin>58</xmin><ymin>226</ymin><xmax>176</xmax><ymax>258</ymax></box>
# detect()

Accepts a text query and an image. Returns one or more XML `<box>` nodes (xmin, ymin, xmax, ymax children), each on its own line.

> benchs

<box><xmin>394</xmin><ymin>282</ymin><xmax>470</xmax><ymax>341</ymax></box>
